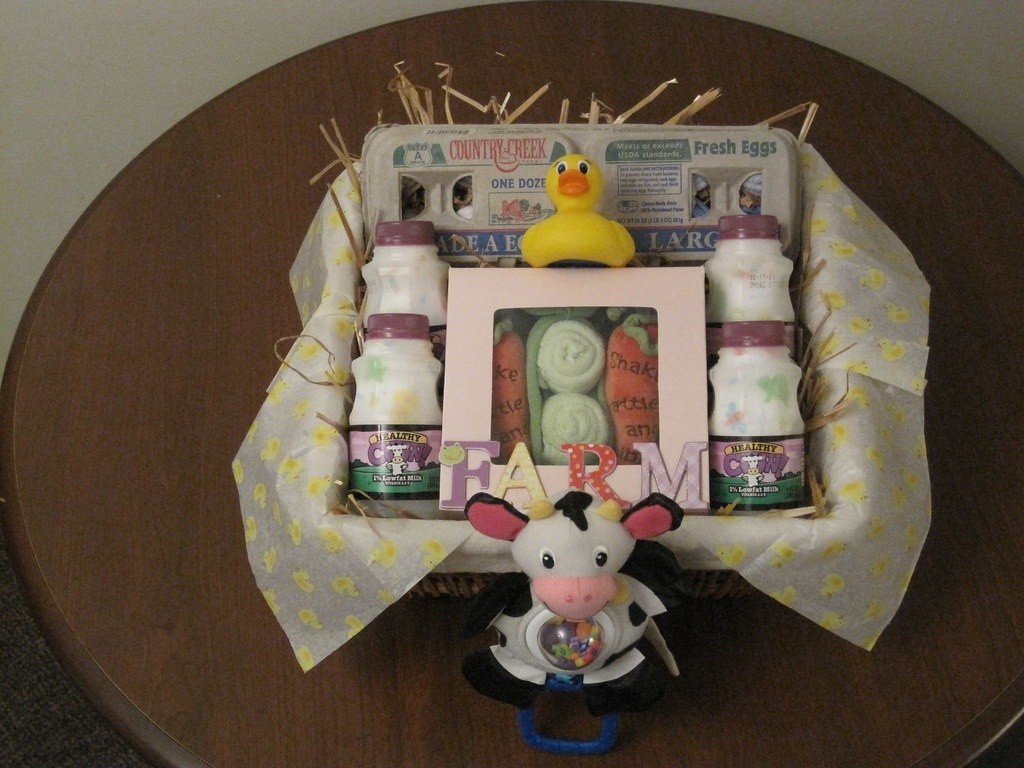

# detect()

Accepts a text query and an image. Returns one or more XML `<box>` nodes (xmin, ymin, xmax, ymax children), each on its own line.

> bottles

<box><xmin>357</xmin><ymin>218</ymin><xmax>448</xmax><ymax>345</ymax></box>
<box><xmin>702</xmin><ymin>215</ymin><xmax>800</xmax><ymax>319</ymax></box>
<box><xmin>346</xmin><ymin>312</ymin><xmax>443</xmax><ymax>522</ymax></box>
<box><xmin>708</xmin><ymin>318</ymin><xmax>807</xmax><ymax>513</ymax></box>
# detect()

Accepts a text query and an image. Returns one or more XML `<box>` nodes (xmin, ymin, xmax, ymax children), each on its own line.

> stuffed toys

<box><xmin>460</xmin><ymin>491</ymin><xmax>685</xmax><ymax>754</ymax></box>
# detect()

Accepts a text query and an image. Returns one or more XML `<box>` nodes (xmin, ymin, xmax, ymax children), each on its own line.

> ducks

<box><xmin>519</xmin><ymin>153</ymin><xmax>638</xmax><ymax>267</ymax></box>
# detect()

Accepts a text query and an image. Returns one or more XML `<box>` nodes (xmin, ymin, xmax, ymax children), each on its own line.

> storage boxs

<box><xmin>436</xmin><ymin>262</ymin><xmax>713</xmax><ymax>518</ymax></box>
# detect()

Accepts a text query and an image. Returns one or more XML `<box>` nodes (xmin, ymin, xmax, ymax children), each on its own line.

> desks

<box><xmin>0</xmin><ymin>0</ymin><xmax>1024</xmax><ymax>768</ymax></box>
